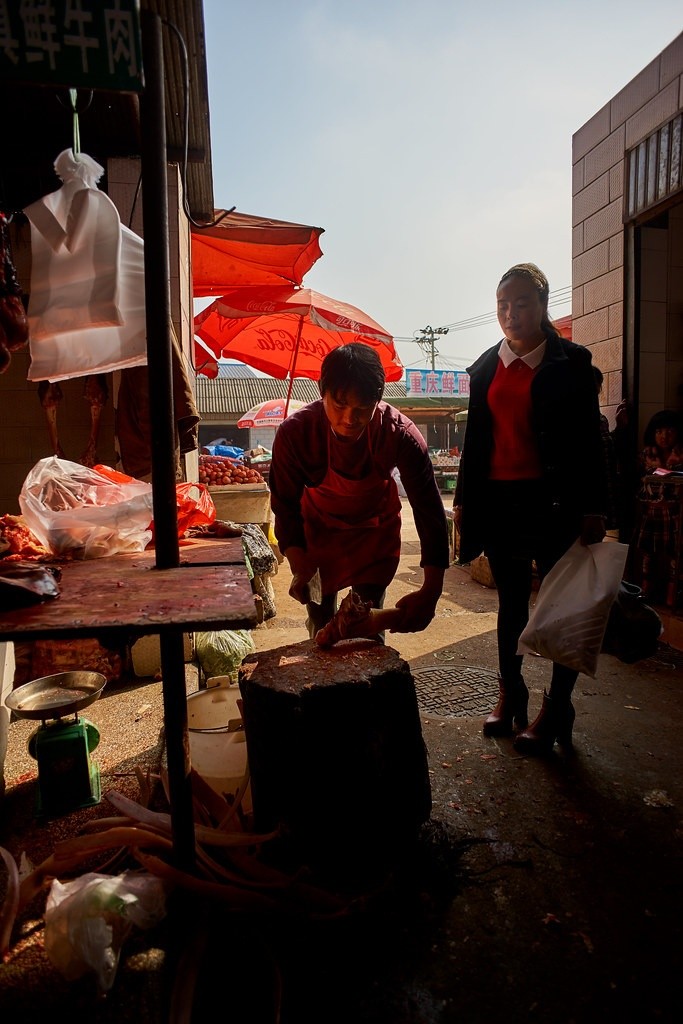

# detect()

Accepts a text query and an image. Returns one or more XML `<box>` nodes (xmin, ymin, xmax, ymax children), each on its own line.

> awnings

<box><xmin>192</xmin><ymin>201</ymin><xmax>326</xmax><ymax>298</ymax></box>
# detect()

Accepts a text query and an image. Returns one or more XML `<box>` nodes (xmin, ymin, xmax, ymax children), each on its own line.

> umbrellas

<box><xmin>237</xmin><ymin>398</ymin><xmax>309</xmax><ymax>440</ymax></box>
<box><xmin>188</xmin><ymin>284</ymin><xmax>402</xmax><ymax>425</ymax></box>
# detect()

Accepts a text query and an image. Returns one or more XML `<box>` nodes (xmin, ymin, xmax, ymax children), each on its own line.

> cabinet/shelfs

<box><xmin>432</xmin><ymin>465</ymin><xmax>460</xmax><ymax>494</ymax></box>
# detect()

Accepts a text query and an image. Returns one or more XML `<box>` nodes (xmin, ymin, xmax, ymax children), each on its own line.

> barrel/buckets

<box><xmin>185</xmin><ymin>676</ymin><xmax>252</xmax><ymax>816</ymax></box>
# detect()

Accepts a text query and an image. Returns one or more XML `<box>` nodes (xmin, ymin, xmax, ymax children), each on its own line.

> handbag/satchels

<box><xmin>601</xmin><ymin>579</ymin><xmax>663</xmax><ymax>665</ymax></box>
<box><xmin>515</xmin><ymin>531</ymin><xmax>630</xmax><ymax>682</ymax></box>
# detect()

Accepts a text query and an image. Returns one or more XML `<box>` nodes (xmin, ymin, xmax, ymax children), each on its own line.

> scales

<box><xmin>4</xmin><ymin>671</ymin><xmax>108</xmax><ymax>808</ymax></box>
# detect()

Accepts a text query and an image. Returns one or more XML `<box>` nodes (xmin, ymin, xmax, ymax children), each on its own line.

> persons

<box><xmin>631</xmin><ymin>411</ymin><xmax>683</xmax><ymax>607</ymax></box>
<box><xmin>453</xmin><ymin>262</ymin><xmax>616</xmax><ymax>754</ymax></box>
<box><xmin>268</xmin><ymin>341</ymin><xmax>449</xmax><ymax>641</ymax></box>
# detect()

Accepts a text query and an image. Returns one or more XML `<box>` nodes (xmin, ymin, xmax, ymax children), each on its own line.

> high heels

<box><xmin>512</xmin><ymin>689</ymin><xmax>576</xmax><ymax>753</ymax></box>
<box><xmin>483</xmin><ymin>669</ymin><xmax>529</xmax><ymax>735</ymax></box>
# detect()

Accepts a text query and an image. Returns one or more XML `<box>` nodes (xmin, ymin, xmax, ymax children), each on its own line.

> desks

<box><xmin>1</xmin><ymin>536</ymin><xmax>260</xmax><ymax>877</ymax></box>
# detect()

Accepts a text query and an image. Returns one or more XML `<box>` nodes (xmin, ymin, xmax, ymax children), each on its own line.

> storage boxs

<box><xmin>469</xmin><ymin>555</ymin><xmax>495</xmax><ymax>587</ymax></box>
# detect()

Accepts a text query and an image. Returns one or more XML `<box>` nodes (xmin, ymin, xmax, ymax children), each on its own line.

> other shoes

<box><xmin>640</xmin><ymin>579</ymin><xmax>656</xmax><ymax>597</ymax></box>
<box><xmin>665</xmin><ymin>582</ymin><xmax>678</xmax><ymax>608</ymax></box>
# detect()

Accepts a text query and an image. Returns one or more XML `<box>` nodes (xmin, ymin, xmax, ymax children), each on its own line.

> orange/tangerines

<box><xmin>200</xmin><ymin>461</ymin><xmax>264</xmax><ymax>485</ymax></box>
